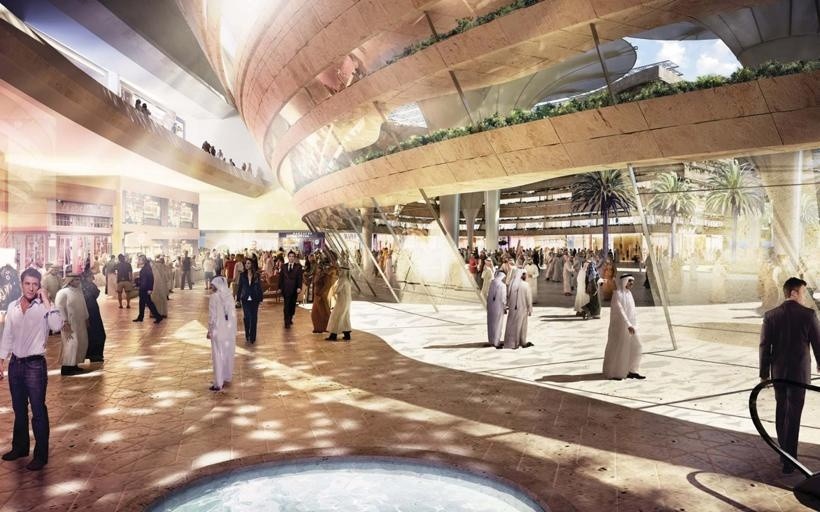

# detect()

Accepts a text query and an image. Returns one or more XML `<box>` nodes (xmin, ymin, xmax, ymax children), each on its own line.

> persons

<box><xmin>758</xmin><ymin>245</ymin><xmax>780</xmax><ymax>318</ymax></box>
<box><xmin>134</xmin><ymin>98</ymin><xmax>152</xmax><ymax>118</ymax></box>
<box><xmin>618</xmin><ymin>239</ymin><xmax>734</xmax><ymax>303</ymax></box>
<box><xmin>201</xmin><ymin>139</ymin><xmax>264</xmax><ymax>179</ymax></box>
<box><xmin>771</xmin><ymin>251</ymin><xmax>800</xmax><ymax>307</ymax></box>
<box><xmin>757</xmin><ymin>277</ymin><xmax>820</xmax><ymax>473</ymax></box>
<box><xmin>458</xmin><ymin>240</ymin><xmax>618</xmax><ymax>350</ymax></box>
<box><xmin>602</xmin><ymin>272</ymin><xmax>647</xmax><ymax>381</ymax></box>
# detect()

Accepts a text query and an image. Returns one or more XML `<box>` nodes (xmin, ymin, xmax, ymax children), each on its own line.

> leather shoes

<box><xmin>153</xmin><ymin>319</ymin><xmax>163</xmax><ymax>323</ymax></box>
<box><xmin>325</xmin><ymin>336</ymin><xmax>336</xmax><ymax>341</ymax></box>
<box><xmin>60</xmin><ymin>366</ymin><xmax>83</xmax><ymax>375</ymax></box>
<box><xmin>133</xmin><ymin>318</ymin><xmax>143</xmax><ymax>322</ymax></box>
<box><xmin>2</xmin><ymin>449</ymin><xmax>29</xmax><ymax>461</ymax></box>
<box><xmin>342</xmin><ymin>336</ymin><xmax>351</xmax><ymax>340</ymax></box>
<box><xmin>27</xmin><ymin>459</ymin><xmax>42</xmax><ymax>470</ymax></box>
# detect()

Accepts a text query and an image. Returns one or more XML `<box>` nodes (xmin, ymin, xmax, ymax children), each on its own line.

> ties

<box><xmin>289</xmin><ymin>264</ymin><xmax>292</xmax><ymax>272</ymax></box>
